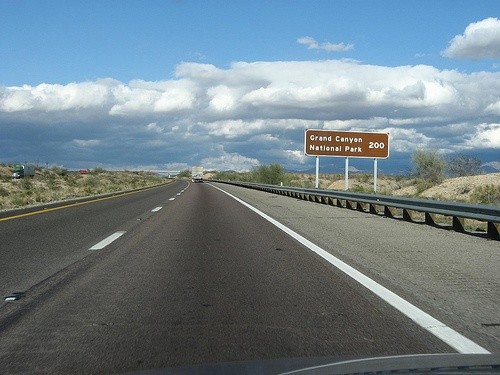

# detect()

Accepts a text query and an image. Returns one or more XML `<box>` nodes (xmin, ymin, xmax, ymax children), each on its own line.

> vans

<box><xmin>80</xmin><ymin>169</ymin><xmax>86</xmax><ymax>174</ymax></box>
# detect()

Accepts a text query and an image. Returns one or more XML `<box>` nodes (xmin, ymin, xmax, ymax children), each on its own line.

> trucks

<box><xmin>11</xmin><ymin>165</ymin><xmax>35</xmax><ymax>179</ymax></box>
<box><xmin>191</xmin><ymin>167</ymin><xmax>203</xmax><ymax>183</ymax></box>
